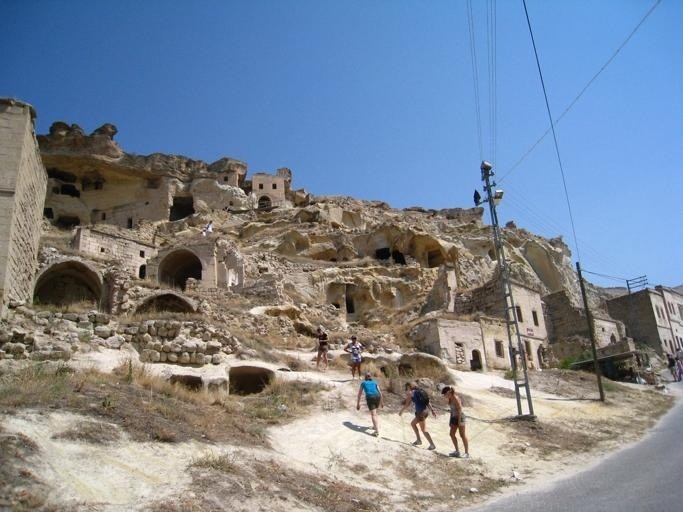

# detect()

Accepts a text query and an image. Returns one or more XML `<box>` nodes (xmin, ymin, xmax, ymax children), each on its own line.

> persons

<box><xmin>344</xmin><ymin>336</ymin><xmax>364</xmax><ymax>380</ymax></box>
<box><xmin>667</xmin><ymin>348</ymin><xmax>683</xmax><ymax>382</ymax></box>
<box><xmin>205</xmin><ymin>221</ymin><xmax>213</xmax><ymax>233</ymax></box>
<box><xmin>357</xmin><ymin>373</ymin><xmax>383</xmax><ymax>436</ymax></box>
<box><xmin>442</xmin><ymin>387</ymin><xmax>469</xmax><ymax>458</ymax></box>
<box><xmin>399</xmin><ymin>383</ymin><xmax>436</xmax><ymax>449</ymax></box>
<box><xmin>316</xmin><ymin>329</ymin><xmax>330</xmax><ymax>370</ymax></box>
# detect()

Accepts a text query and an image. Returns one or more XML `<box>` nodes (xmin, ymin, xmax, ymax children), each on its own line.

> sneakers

<box><xmin>369</xmin><ymin>425</ymin><xmax>374</xmax><ymax>429</ymax></box>
<box><xmin>449</xmin><ymin>451</ymin><xmax>458</xmax><ymax>456</ymax></box>
<box><xmin>413</xmin><ymin>441</ymin><xmax>422</xmax><ymax>445</ymax></box>
<box><xmin>427</xmin><ymin>445</ymin><xmax>435</xmax><ymax>450</ymax></box>
<box><xmin>460</xmin><ymin>452</ymin><xmax>469</xmax><ymax>458</ymax></box>
<box><xmin>372</xmin><ymin>431</ymin><xmax>379</xmax><ymax>435</ymax></box>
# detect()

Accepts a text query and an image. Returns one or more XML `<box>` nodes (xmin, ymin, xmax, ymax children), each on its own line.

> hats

<box><xmin>441</xmin><ymin>387</ymin><xmax>449</xmax><ymax>394</ymax></box>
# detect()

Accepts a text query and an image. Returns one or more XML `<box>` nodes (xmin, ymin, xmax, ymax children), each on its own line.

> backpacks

<box><xmin>410</xmin><ymin>385</ymin><xmax>428</xmax><ymax>408</ymax></box>
